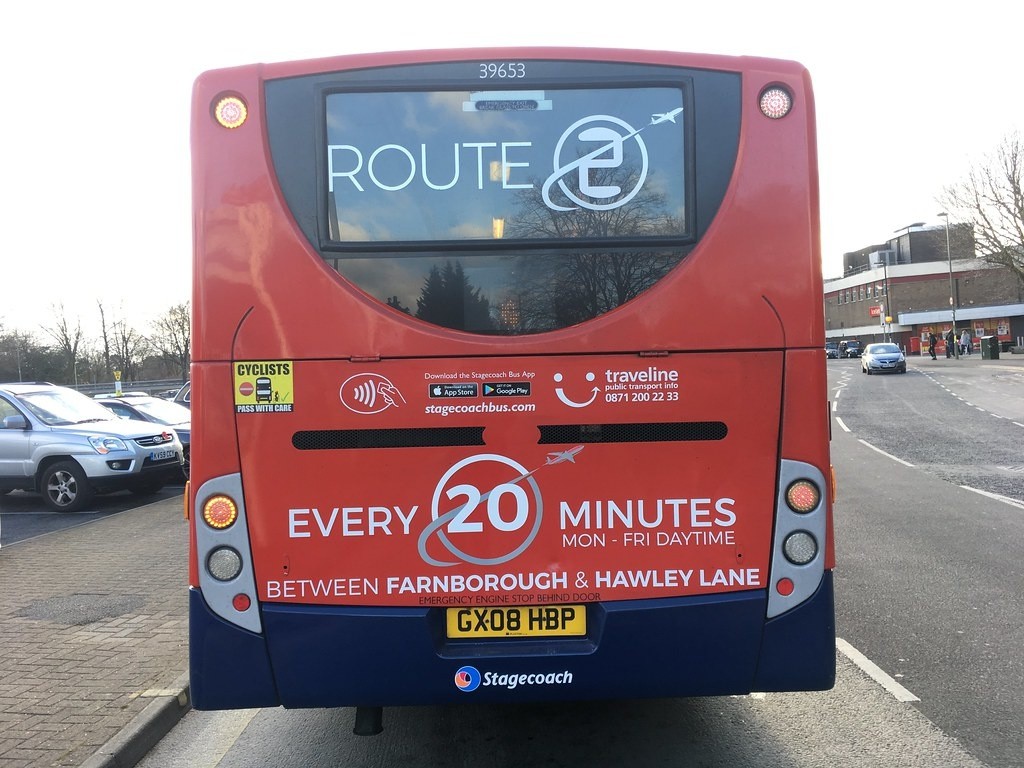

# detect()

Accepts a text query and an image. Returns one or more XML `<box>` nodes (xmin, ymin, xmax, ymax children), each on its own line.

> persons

<box><xmin>946</xmin><ymin>328</ymin><xmax>955</xmax><ymax>356</ymax></box>
<box><xmin>928</xmin><ymin>333</ymin><xmax>937</xmax><ymax>360</ymax></box>
<box><xmin>959</xmin><ymin>329</ymin><xmax>971</xmax><ymax>355</ymax></box>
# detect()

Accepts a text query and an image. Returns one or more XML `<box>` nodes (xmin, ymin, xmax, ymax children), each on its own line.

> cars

<box><xmin>861</xmin><ymin>343</ymin><xmax>907</xmax><ymax>376</ymax></box>
<box><xmin>837</xmin><ymin>340</ymin><xmax>864</xmax><ymax>359</ymax></box>
<box><xmin>825</xmin><ymin>341</ymin><xmax>837</xmax><ymax>359</ymax></box>
<box><xmin>174</xmin><ymin>381</ymin><xmax>191</xmax><ymax>410</ymax></box>
<box><xmin>94</xmin><ymin>395</ymin><xmax>191</xmax><ymax>485</ymax></box>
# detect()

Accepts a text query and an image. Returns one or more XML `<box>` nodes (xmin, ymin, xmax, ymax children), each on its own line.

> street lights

<box><xmin>874</xmin><ymin>263</ymin><xmax>891</xmax><ymax>343</ymax></box>
<box><xmin>938</xmin><ymin>213</ymin><xmax>958</xmax><ymax>359</ymax></box>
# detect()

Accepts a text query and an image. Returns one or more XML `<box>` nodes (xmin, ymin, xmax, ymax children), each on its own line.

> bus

<box><xmin>181</xmin><ymin>45</ymin><xmax>835</xmax><ymax>735</ymax></box>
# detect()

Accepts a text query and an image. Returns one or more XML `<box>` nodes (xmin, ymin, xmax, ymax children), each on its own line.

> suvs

<box><xmin>0</xmin><ymin>382</ymin><xmax>184</xmax><ymax>513</ymax></box>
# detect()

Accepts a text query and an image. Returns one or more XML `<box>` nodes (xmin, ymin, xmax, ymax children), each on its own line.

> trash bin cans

<box><xmin>981</xmin><ymin>336</ymin><xmax>1000</xmax><ymax>360</ymax></box>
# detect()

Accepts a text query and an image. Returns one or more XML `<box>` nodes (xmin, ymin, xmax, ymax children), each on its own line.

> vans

<box><xmin>94</xmin><ymin>392</ymin><xmax>150</xmax><ymax>398</ymax></box>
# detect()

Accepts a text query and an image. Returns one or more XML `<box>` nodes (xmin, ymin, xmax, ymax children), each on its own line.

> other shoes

<box><xmin>932</xmin><ymin>355</ymin><xmax>937</xmax><ymax>360</ymax></box>
